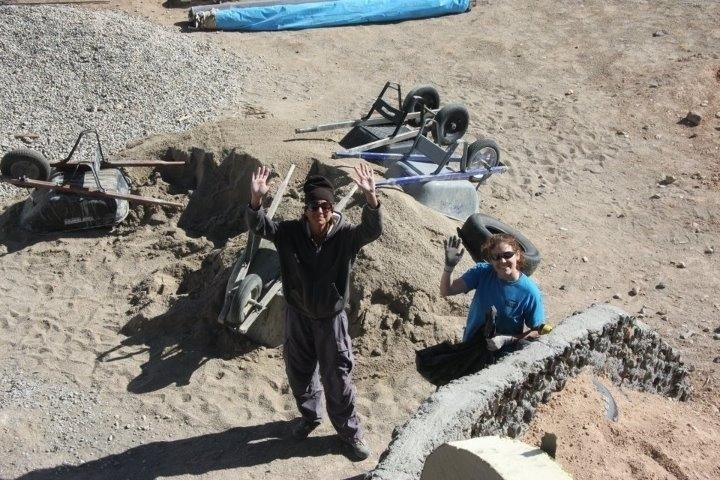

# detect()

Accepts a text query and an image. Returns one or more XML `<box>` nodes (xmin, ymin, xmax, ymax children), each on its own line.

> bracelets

<box><xmin>444</xmin><ymin>265</ymin><xmax>455</xmax><ymax>273</ymax></box>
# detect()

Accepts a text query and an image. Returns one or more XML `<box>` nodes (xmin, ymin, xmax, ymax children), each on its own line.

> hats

<box><xmin>304</xmin><ymin>188</ymin><xmax>332</xmax><ymax>203</ymax></box>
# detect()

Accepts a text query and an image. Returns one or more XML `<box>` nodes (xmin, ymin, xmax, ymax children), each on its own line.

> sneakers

<box><xmin>293</xmin><ymin>419</ymin><xmax>315</xmax><ymax>440</ymax></box>
<box><xmin>352</xmin><ymin>438</ymin><xmax>371</xmax><ymax>461</ymax></box>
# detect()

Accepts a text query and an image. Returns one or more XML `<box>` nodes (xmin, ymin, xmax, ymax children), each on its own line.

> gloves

<box><xmin>443</xmin><ymin>235</ymin><xmax>465</xmax><ymax>273</ymax></box>
<box><xmin>486</xmin><ymin>335</ymin><xmax>515</xmax><ymax>352</ymax></box>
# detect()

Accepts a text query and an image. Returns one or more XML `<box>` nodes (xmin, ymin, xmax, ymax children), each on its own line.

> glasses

<box><xmin>308</xmin><ymin>201</ymin><xmax>332</xmax><ymax>211</ymax></box>
<box><xmin>491</xmin><ymin>251</ymin><xmax>515</xmax><ymax>261</ymax></box>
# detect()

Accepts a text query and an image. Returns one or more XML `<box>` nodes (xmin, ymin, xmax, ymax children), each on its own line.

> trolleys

<box><xmin>331</xmin><ymin>117</ymin><xmax>508</xmax><ymax>225</ymax></box>
<box><xmin>293</xmin><ymin>80</ymin><xmax>472</xmax><ymax>155</ymax></box>
<box><xmin>1</xmin><ymin>126</ymin><xmax>191</xmax><ymax>235</ymax></box>
<box><xmin>216</xmin><ymin>160</ymin><xmax>359</xmax><ymax>349</ymax></box>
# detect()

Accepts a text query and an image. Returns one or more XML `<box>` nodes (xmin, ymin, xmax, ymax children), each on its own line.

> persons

<box><xmin>438</xmin><ymin>232</ymin><xmax>545</xmax><ymax>354</ymax></box>
<box><xmin>244</xmin><ymin>163</ymin><xmax>383</xmax><ymax>460</ymax></box>
